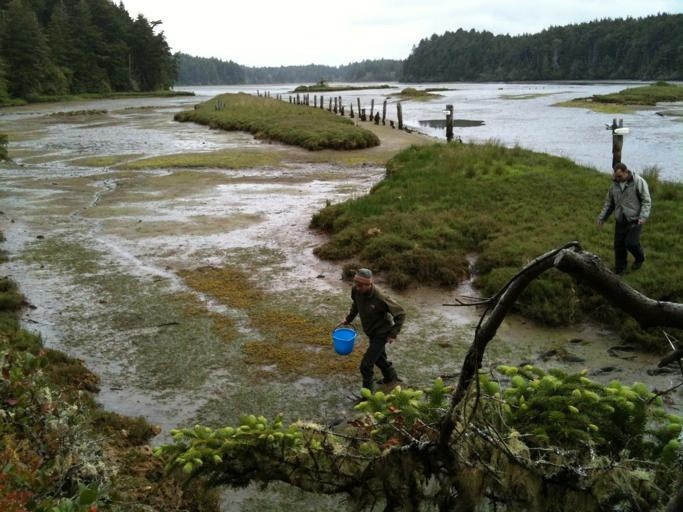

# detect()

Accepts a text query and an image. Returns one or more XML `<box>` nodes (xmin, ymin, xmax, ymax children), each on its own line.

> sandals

<box><xmin>378</xmin><ymin>372</ymin><xmax>397</xmax><ymax>381</ymax></box>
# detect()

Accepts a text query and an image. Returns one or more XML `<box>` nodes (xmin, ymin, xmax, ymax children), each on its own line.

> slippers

<box><xmin>631</xmin><ymin>254</ymin><xmax>645</xmax><ymax>270</ymax></box>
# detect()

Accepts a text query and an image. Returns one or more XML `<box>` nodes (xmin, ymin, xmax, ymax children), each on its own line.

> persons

<box><xmin>340</xmin><ymin>267</ymin><xmax>407</xmax><ymax>399</ymax></box>
<box><xmin>596</xmin><ymin>161</ymin><xmax>653</xmax><ymax>274</ymax></box>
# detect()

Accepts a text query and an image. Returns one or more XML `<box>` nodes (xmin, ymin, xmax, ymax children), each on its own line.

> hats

<box><xmin>353</xmin><ymin>269</ymin><xmax>372</xmax><ymax>285</ymax></box>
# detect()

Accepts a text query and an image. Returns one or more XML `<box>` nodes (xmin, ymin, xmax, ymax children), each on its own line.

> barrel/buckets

<box><xmin>332</xmin><ymin>322</ymin><xmax>357</xmax><ymax>355</ymax></box>
